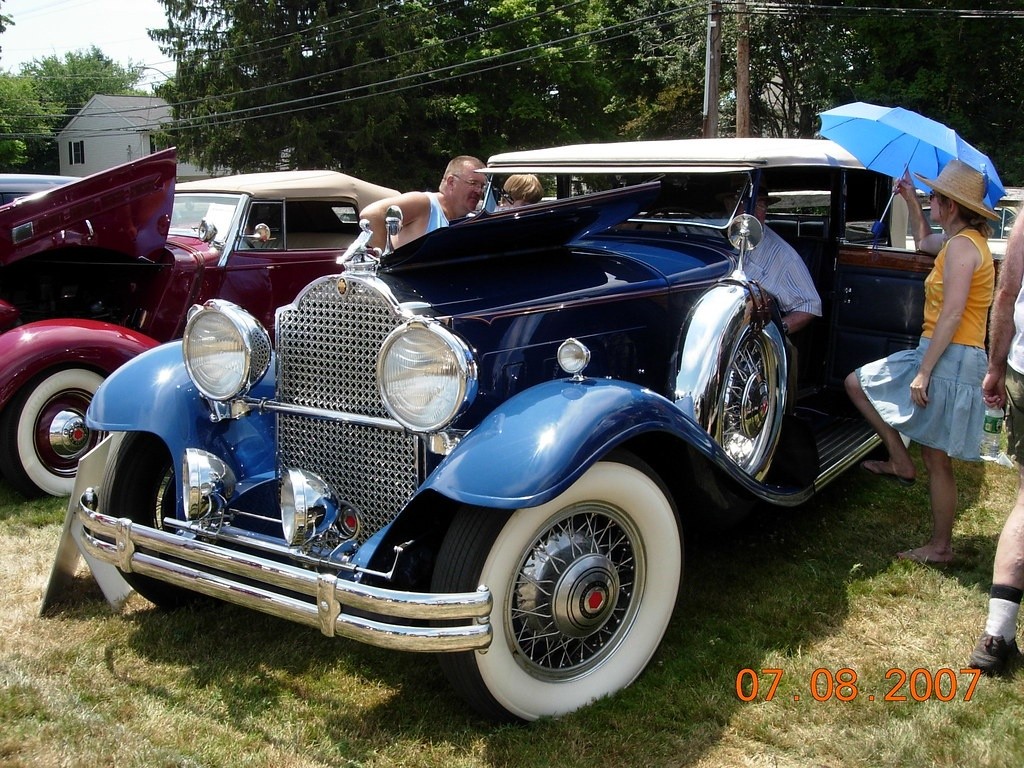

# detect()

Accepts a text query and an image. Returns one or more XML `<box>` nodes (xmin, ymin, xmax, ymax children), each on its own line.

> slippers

<box><xmin>860</xmin><ymin>459</ymin><xmax>916</xmax><ymax>486</ymax></box>
<box><xmin>896</xmin><ymin>549</ymin><xmax>949</xmax><ymax>569</ymax></box>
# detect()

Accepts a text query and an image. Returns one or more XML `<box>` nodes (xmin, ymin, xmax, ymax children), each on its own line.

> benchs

<box><xmin>237</xmin><ymin>231</ymin><xmax>360</xmax><ymax>252</ymax></box>
<box><xmin>823</xmin><ymin>248</ymin><xmax>942</xmax><ymax>394</ymax></box>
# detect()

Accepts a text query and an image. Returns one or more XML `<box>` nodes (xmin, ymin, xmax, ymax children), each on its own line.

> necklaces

<box><xmin>947</xmin><ymin>224</ymin><xmax>969</xmax><ymax>241</ymax></box>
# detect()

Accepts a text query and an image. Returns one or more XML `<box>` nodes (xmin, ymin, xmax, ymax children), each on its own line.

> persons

<box><xmin>495</xmin><ymin>174</ymin><xmax>544</xmax><ymax>212</ymax></box>
<box><xmin>968</xmin><ymin>206</ymin><xmax>1024</xmax><ymax>672</ymax></box>
<box><xmin>359</xmin><ymin>156</ymin><xmax>487</xmax><ymax>254</ymax></box>
<box><xmin>845</xmin><ymin>160</ymin><xmax>1002</xmax><ymax>561</ymax></box>
<box><xmin>712</xmin><ymin>190</ymin><xmax>822</xmax><ymax>415</ymax></box>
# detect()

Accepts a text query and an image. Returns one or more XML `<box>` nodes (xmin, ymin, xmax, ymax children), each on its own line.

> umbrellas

<box><xmin>819</xmin><ymin>102</ymin><xmax>1007</xmax><ymax>236</ymax></box>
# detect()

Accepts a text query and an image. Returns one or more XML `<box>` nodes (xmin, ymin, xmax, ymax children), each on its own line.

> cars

<box><xmin>0</xmin><ymin>167</ymin><xmax>403</xmax><ymax>508</ymax></box>
<box><xmin>69</xmin><ymin>134</ymin><xmax>1002</xmax><ymax>730</ymax></box>
<box><xmin>898</xmin><ymin>203</ymin><xmax>1021</xmax><ymax>265</ymax></box>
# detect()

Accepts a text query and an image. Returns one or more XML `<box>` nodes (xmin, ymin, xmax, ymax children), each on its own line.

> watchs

<box><xmin>781</xmin><ymin>320</ymin><xmax>788</xmax><ymax>334</ymax></box>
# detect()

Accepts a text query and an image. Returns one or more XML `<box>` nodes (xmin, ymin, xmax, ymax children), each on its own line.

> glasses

<box><xmin>732</xmin><ymin>199</ymin><xmax>768</xmax><ymax>216</ymax></box>
<box><xmin>929</xmin><ymin>191</ymin><xmax>942</xmax><ymax>200</ymax></box>
<box><xmin>450</xmin><ymin>174</ymin><xmax>486</xmax><ymax>192</ymax></box>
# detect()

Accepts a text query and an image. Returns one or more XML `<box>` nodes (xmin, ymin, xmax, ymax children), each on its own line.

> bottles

<box><xmin>980</xmin><ymin>403</ymin><xmax>1005</xmax><ymax>461</ymax></box>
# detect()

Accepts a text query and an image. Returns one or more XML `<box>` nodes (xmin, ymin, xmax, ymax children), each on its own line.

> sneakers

<box><xmin>969</xmin><ymin>631</ymin><xmax>1024</xmax><ymax>671</ymax></box>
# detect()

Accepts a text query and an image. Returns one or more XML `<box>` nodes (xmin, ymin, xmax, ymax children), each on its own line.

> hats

<box><xmin>715</xmin><ymin>172</ymin><xmax>781</xmax><ymax>206</ymax></box>
<box><xmin>912</xmin><ymin>160</ymin><xmax>1001</xmax><ymax>222</ymax></box>
